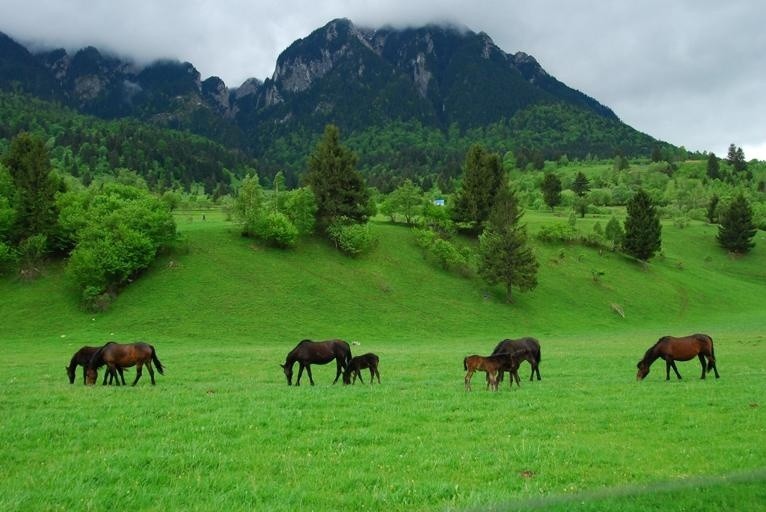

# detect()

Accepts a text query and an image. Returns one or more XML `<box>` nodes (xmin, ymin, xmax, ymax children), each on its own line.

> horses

<box><xmin>279</xmin><ymin>338</ymin><xmax>353</xmax><ymax>386</ymax></box>
<box><xmin>636</xmin><ymin>333</ymin><xmax>720</xmax><ymax>383</ymax></box>
<box><xmin>64</xmin><ymin>345</ymin><xmax>128</xmax><ymax>387</ymax></box>
<box><xmin>339</xmin><ymin>352</ymin><xmax>382</xmax><ymax>386</ymax></box>
<box><xmin>464</xmin><ymin>336</ymin><xmax>542</xmax><ymax>392</ymax></box>
<box><xmin>84</xmin><ymin>340</ymin><xmax>164</xmax><ymax>387</ymax></box>
